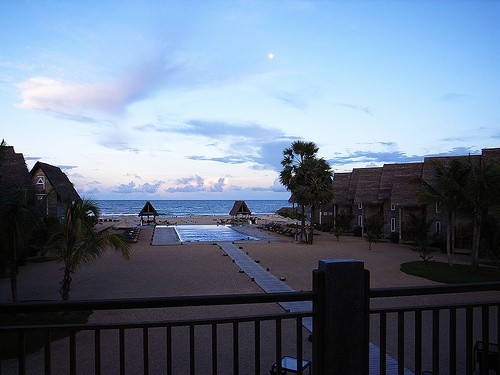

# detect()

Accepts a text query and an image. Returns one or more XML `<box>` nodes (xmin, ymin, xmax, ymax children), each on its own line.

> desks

<box><xmin>471</xmin><ymin>338</ymin><xmax>500</xmax><ymax>372</ymax></box>
<box><xmin>270</xmin><ymin>356</ymin><xmax>311</xmax><ymax>375</ymax></box>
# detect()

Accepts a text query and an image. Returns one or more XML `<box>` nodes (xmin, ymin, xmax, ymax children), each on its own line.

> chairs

<box><xmin>120</xmin><ymin>227</ymin><xmax>141</xmax><ymax>243</ymax></box>
<box><xmin>258</xmin><ymin>220</ymin><xmax>307</xmax><ymax>237</ymax></box>
